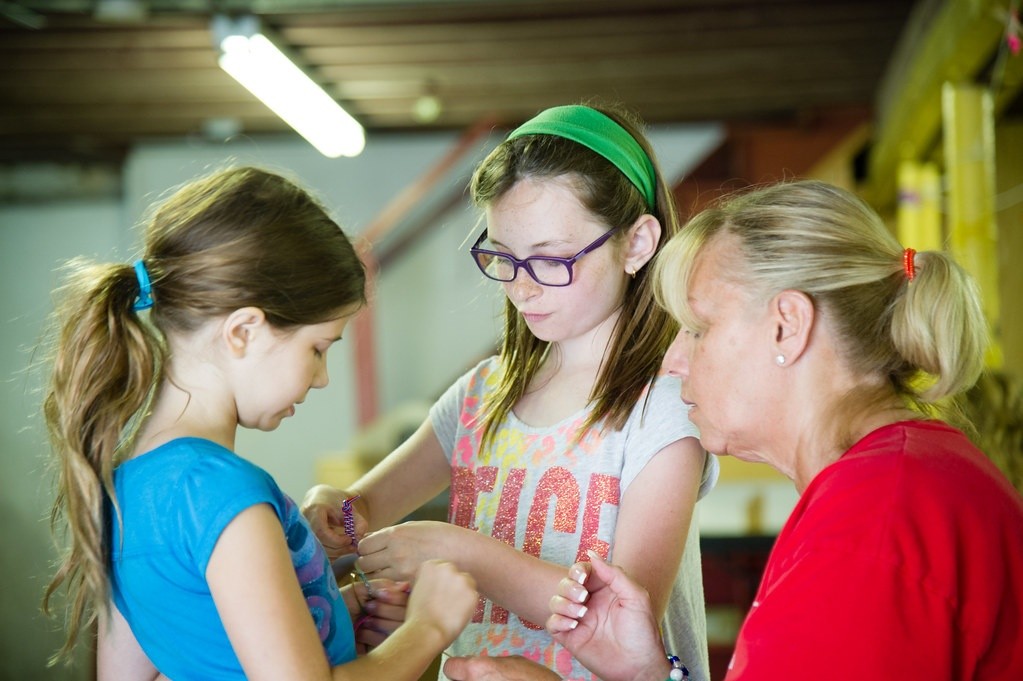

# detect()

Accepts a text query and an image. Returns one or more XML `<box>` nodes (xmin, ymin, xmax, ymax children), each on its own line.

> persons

<box><xmin>649</xmin><ymin>180</ymin><xmax>1022</xmax><ymax>681</ymax></box>
<box><xmin>40</xmin><ymin>166</ymin><xmax>479</xmax><ymax>681</ymax></box>
<box><xmin>300</xmin><ymin>105</ymin><xmax>722</xmax><ymax>681</ymax></box>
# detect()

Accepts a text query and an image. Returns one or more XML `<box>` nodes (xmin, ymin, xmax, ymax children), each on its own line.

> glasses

<box><xmin>468</xmin><ymin>213</ymin><xmax>639</xmax><ymax>289</ymax></box>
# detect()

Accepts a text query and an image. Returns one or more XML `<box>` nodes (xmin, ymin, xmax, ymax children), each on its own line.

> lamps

<box><xmin>207</xmin><ymin>16</ymin><xmax>369</xmax><ymax>161</ymax></box>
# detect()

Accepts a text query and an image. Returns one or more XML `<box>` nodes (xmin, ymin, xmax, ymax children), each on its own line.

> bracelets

<box><xmin>664</xmin><ymin>653</ymin><xmax>690</xmax><ymax>681</ymax></box>
